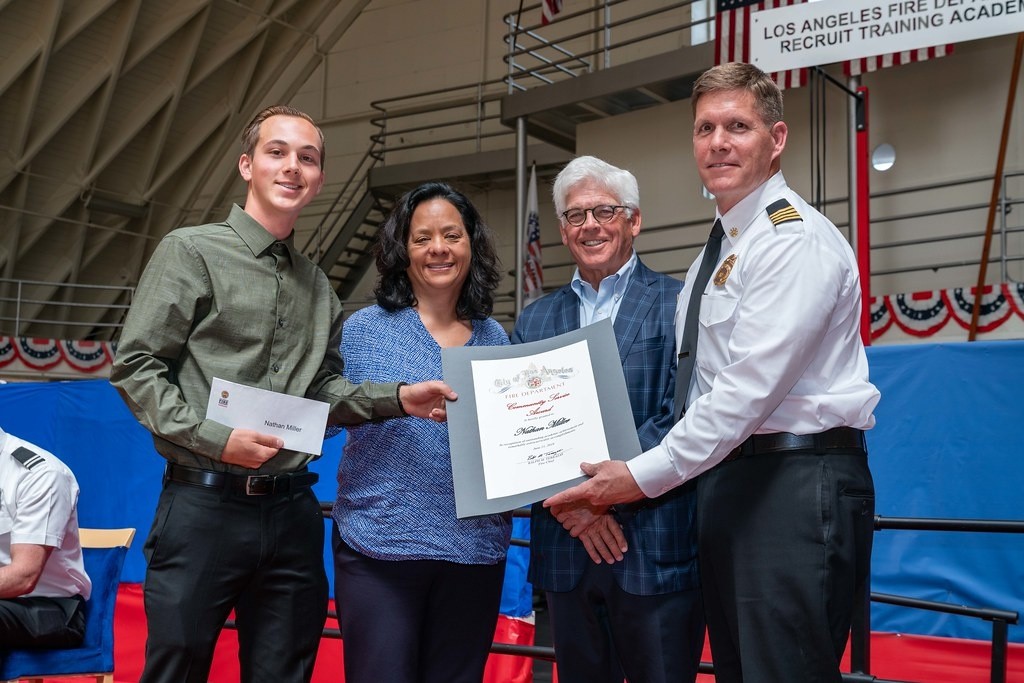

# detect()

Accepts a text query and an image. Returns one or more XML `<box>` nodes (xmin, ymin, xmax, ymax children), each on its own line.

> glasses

<box><xmin>562</xmin><ymin>202</ymin><xmax>632</xmax><ymax>228</ymax></box>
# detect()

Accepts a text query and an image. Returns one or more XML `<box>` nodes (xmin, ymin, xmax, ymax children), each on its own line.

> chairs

<box><xmin>0</xmin><ymin>528</ymin><xmax>136</xmax><ymax>683</ymax></box>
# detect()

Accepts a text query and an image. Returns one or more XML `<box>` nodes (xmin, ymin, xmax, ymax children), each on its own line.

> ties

<box><xmin>674</xmin><ymin>217</ymin><xmax>724</xmax><ymax>422</ymax></box>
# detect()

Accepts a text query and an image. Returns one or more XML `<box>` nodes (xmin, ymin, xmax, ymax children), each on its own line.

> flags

<box><xmin>713</xmin><ymin>0</ymin><xmax>808</xmax><ymax>95</ymax></box>
<box><xmin>522</xmin><ymin>166</ymin><xmax>543</xmax><ymax>307</ymax></box>
<box><xmin>844</xmin><ymin>37</ymin><xmax>957</xmax><ymax>77</ymax></box>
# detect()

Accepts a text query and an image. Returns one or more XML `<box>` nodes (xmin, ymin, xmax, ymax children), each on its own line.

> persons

<box><xmin>543</xmin><ymin>62</ymin><xmax>881</xmax><ymax>683</ymax></box>
<box><xmin>110</xmin><ymin>104</ymin><xmax>457</xmax><ymax>683</ymax></box>
<box><xmin>0</xmin><ymin>429</ymin><xmax>92</xmax><ymax>683</ymax></box>
<box><xmin>511</xmin><ymin>153</ymin><xmax>707</xmax><ymax>683</ymax></box>
<box><xmin>328</xmin><ymin>182</ymin><xmax>513</xmax><ymax>683</ymax></box>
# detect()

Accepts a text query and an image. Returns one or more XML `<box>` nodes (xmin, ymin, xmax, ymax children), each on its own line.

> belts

<box><xmin>727</xmin><ymin>429</ymin><xmax>864</xmax><ymax>459</ymax></box>
<box><xmin>161</xmin><ymin>462</ymin><xmax>320</xmax><ymax>499</ymax></box>
